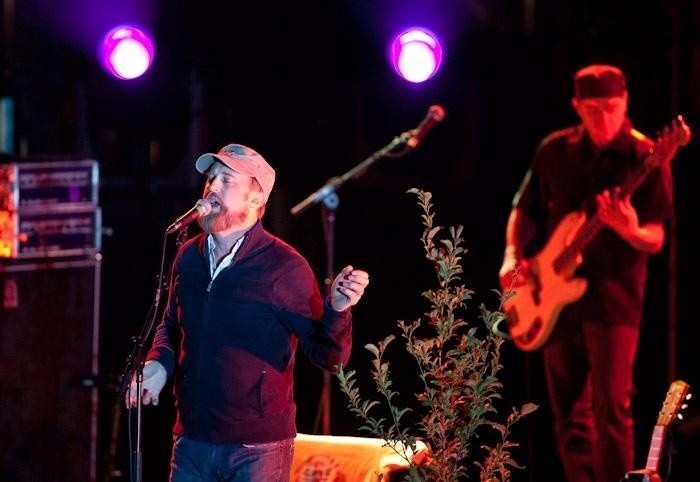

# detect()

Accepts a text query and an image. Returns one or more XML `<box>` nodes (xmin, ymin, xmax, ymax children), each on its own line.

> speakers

<box><xmin>1</xmin><ymin>257</ymin><xmax>101</xmax><ymax>478</ymax></box>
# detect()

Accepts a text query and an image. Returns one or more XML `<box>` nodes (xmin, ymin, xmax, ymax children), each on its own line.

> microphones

<box><xmin>169</xmin><ymin>199</ymin><xmax>212</xmax><ymax>232</ymax></box>
<box><xmin>409</xmin><ymin>105</ymin><xmax>445</xmax><ymax>147</ymax></box>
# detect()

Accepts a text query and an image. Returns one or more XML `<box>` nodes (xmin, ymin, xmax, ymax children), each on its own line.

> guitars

<box><xmin>502</xmin><ymin>116</ymin><xmax>691</xmax><ymax>352</ymax></box>
<box><xmin>642</xmin><ymin>380</ymin><xmax>692</xmax><ymax>478</ymax></box>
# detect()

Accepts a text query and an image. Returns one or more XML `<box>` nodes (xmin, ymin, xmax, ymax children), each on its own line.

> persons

<box><xmin>499</xmin><ymin>64</ymin><xmax>674</xmax><ymax>482</ymax></box>
<box><xmin>124</xmin><ymin>142</ymin><xmax>369</xmax><ymax>482</ymax></box>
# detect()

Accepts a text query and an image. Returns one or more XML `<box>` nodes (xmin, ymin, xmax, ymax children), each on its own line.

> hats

<box><xmin>571</xmin><ymin>61</ymin><xmax>631</xmax><ymax>102</ymax></box>
<box><xmin>192</xmin><ymin>140</ymin><xmax>280</xmax><ymax>205</ymax></box>
